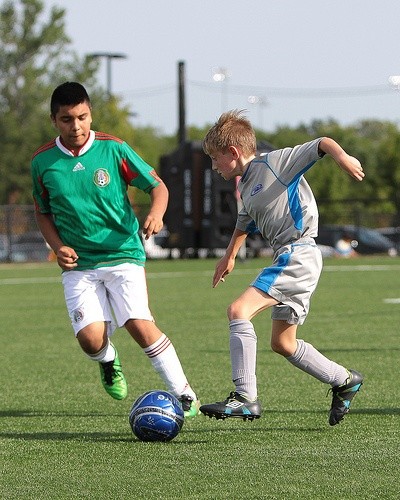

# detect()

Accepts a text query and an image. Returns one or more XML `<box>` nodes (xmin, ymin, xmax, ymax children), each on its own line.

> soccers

<box><xmin>129</xmin><ymin>391</ymin><xmax>184</xmax><ymax>443</ymax></box>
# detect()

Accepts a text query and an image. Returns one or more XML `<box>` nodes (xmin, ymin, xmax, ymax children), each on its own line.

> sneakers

<box><xmin>98</xmin><ymin>342</ymin><xmax>128</xmax><ymax>400</ymax></box>
<box><xmin>326</xmin><ymin>369</ymin><xmax>363</xmax><ymax>426</ymax></box>
<box><xmin>199</xmin><ymin>391</ymin><xmax>262</xmax><ymax>422</ymax></box>
<box><xmin>178</xmin><ymin>392</ymin><xmax>202</xmax><ymax>418</ymax></box>
